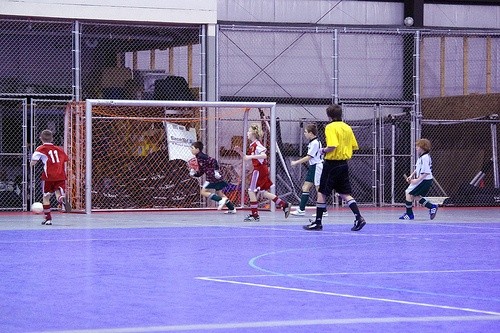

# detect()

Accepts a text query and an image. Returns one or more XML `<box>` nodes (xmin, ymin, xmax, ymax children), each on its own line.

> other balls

<box><xmin>31</xmin><ymin>202</ymin><xmax>44</xmax><ymax>214</ymax></box>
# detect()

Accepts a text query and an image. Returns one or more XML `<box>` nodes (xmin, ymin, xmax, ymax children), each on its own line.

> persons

<box><xmin>302</xmin><ymin>103</ymin><xmax>367</xmax><ymax>233</ymax></box>
<box><xmin>29</xmin><ymin>129</ymin><xmax>74</xmax><ymax>226</ymax></box>
<box><xmin>184</xmin><ymin>139</ymin><xmax>237</xmax><ymax>215</ymax></box>
<box><xmin>290</xmin><ymin>121</ymin><xmax>331</xmax><ymax>218</ymax></box>
<box><xmin>399</xmin><ymin>136</ymin><xmax>442</xmax><ymax>222</ymax></box>
<box><xmin>232</xmin><ymin>121</ymin><xmax>293</xmax><ymax>223</ymax></box>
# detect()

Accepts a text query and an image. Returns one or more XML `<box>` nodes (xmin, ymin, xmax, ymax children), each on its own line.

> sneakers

<box><xmin>41</xmin><ymin>218</ymin><xmax>52</xmax><ymax>225</ymax></box>
<box><xmin>242</xmin><ymin>214</ymin><xmax>260</xmax><ymax>222</ymax></box>
<box><xmin>289</xmin><ymin>208</ymin><xmax>306</xmax><ymax>216</ymax></box>
<box><xmin>399</xmin><ymin>213</ymin><xmax>414</xmax><ymax>219</ymax></box>
<box><xmin>351</xmin><ymin>217</ymin><xmax>366</xmax><ymax>231</ymax></box>
<box><xmin>224</xmin><ymin>208</ymin><xmax>237</xmax><ymax>214</ymax></box>
<box><xmin>60</xmin><ymin>197</ymin><xmax>71</xmax><ymax>213</ymax></box>
<box><xmin>283</xmin><ymin>202</ymin><xmax>293</xmax><ymax>219</ymax></box>
<box><xmin>312</xmin><ymin>211</ymin><xmax>328</xmax><ymax>217</ymax></box>
<box><xmin>218</xmin><ymin>196</ymin><xmax>227</xmax><ymax>211</ymax></box>
<box><xmin>430</xmin><ymin>205</ymin><xmax>438</xmax><ymax>220</ymax></box>
<box><xmin>303</xmin><ymin>222</ymin><xmax>323</xmax><ymax>231</ymax></box>
